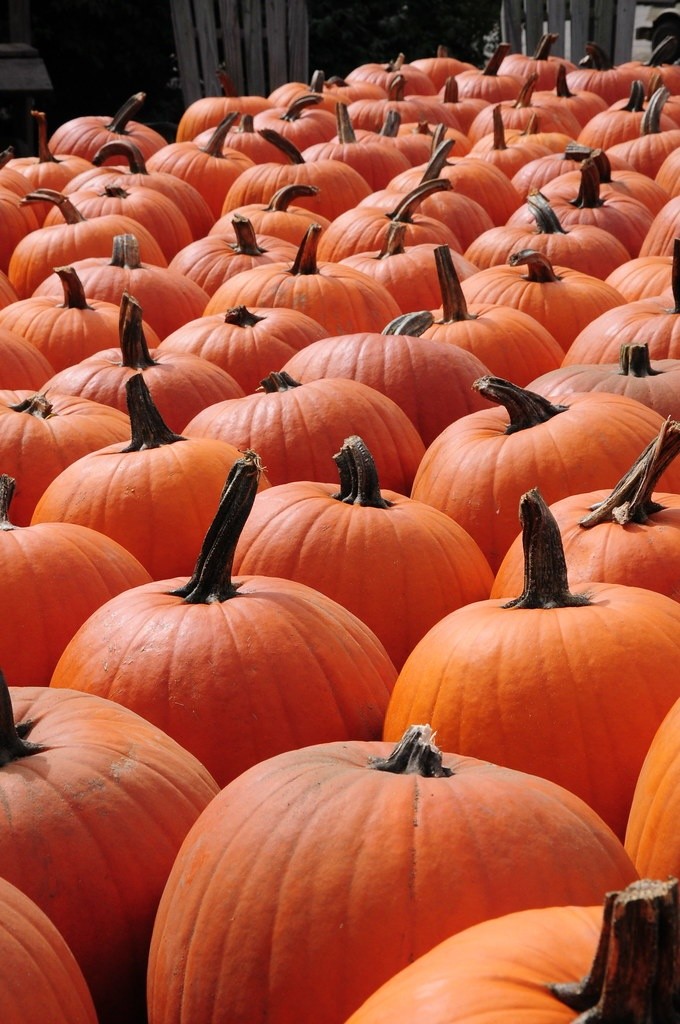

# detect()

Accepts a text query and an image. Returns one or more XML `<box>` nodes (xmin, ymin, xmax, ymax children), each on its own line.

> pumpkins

<box><xmin>0</xmin><ymin>33</ymin><xmax>680</xmax><ymax>1024</ymax></box>
